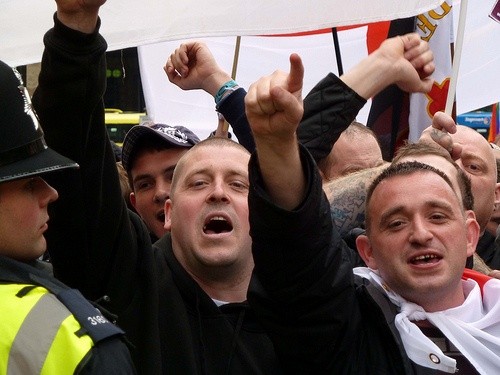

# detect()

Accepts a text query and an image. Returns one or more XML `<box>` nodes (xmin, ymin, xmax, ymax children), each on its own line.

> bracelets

<box><xmin>214</xmin><ymin>79</ymin><xmax>240</xmax><ymax>104</ymax></box>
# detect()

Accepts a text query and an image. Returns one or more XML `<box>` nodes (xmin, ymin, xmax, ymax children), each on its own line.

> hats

<box><xmin>122</xmin><ymin>124</ymin><xmax>200</xmax><ymax>175</ymax></box>
<box><xmin>0</xmin><ymin>61</ymin><xmax>80</xmax><ymax>183</ymax></box>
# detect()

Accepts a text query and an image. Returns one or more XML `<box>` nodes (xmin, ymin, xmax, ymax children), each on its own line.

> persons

<box><xmin>31</xmin><ymin>0</ymin><xmax>500</xmax><ymax>375</ymax></box>
<box><xmin>245</xmin><ymin>33</ymin><xmax>500</xmax><ymax>375</ymax></box>
<box><xmin>0</xmin><ymin>59</ymin><xmax>137</xmax><ymax>375</ymax></box>
<box><xmin>32</xmin><ymin>0</ymin><xmax>255</xmax><ymax>374</ymax></box>
